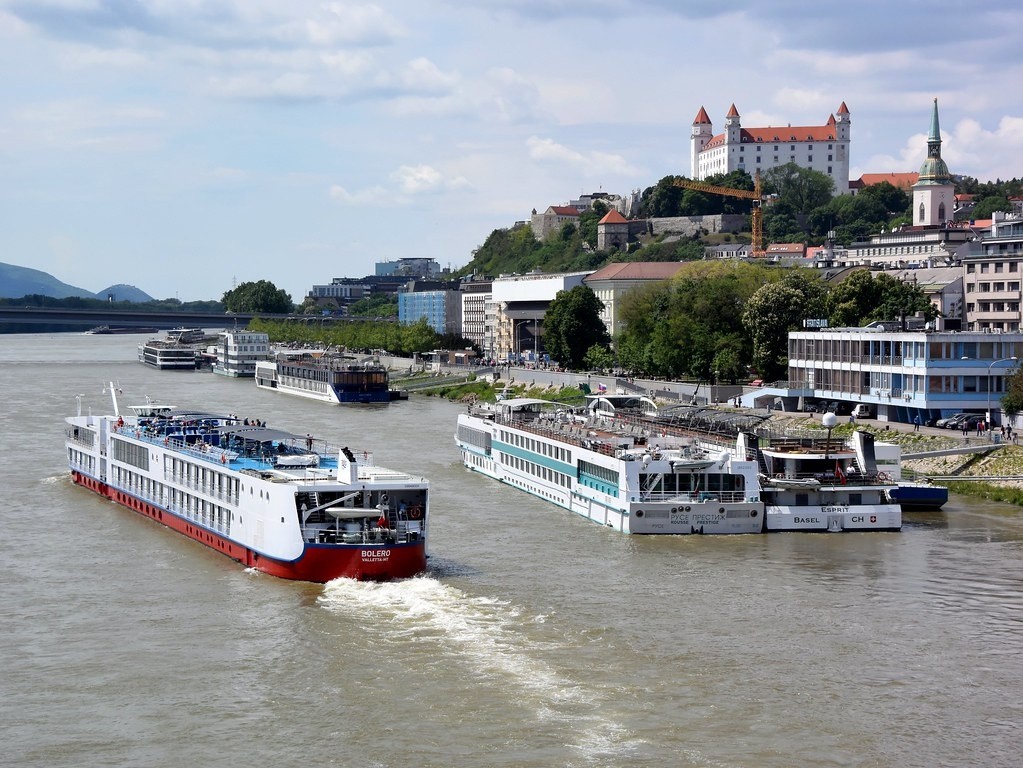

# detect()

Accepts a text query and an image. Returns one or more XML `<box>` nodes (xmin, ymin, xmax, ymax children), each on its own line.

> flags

<box><xmin>835</xmin><ymin>461</ymin><xmax>846</xmax><ymax>484</ymax></box>
<box><xmin>599</xmin><ymin>384</ymin><xmax>606</xmax><ymax>391</ymax></box>
<box><xmin>694</xmin><ymin>474</ymin><xmax>699</xmax><ymax>495</ymax></box>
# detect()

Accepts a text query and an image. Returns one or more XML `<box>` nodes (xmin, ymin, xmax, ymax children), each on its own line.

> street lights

<box><xmin>298</xmin><ymin>316</ymin><xmax>333</xmax><ymax>326</ymax></box>
<box><xmin>961</xmin><ymin>357</ymin><xmax>1017</xmax><ymax>433</ymax></box>
<box><xmin>516</xmin><ymin>320</ymin><xmax>530</xmax><ymax>360</ymax></box>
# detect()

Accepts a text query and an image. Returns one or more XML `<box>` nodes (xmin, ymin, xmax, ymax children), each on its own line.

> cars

<box><xmin>804</xmin><ymin>400</ymin><xmax>818</xmax><ymax>412</ymax></box>
<box><xmin>826</xmin><ymin>402</ymin><xmax>848</xmax><ymax>415</ymax></box>
<box><xmin>945</xmin><ymin>413</ymin><xmax>985</xmax><ymax>430</ymax></box>
<box><xmin>958</xmin><ymin>416</ymin><xmax>997</xmax><ymax>431</ymax></box>
<box><xmin>936</xmin><ymin>413</ymin><xmax>966</xmax><ymax>429</ymax></box>
<box><xmin>925</xmin><ymin>416</ymin><xmax>942</xmax><ymax>427</ymax></box>
<box><xmin>850</xmin><ymin>404</ymin><xmax>871</xmax><ymax>419</ymax></box>
<box><xmin>498</xmin><ymin>358</ymin><xmax>508</xmax><ymax>366</ymax></box>
<box><xmin>815</xmin><ymin>401</ymin><xmax>831</xmax><ymax>414</ymax></box>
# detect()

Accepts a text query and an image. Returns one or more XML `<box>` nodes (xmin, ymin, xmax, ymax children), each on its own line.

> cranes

<box><xmin>672</xmin><ymin>167</ymin><xmax>781</xmax><ymax>257</ymax></box>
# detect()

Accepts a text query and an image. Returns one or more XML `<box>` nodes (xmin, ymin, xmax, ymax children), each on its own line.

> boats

<box><xmin>768</xmin><ymin>479</ymin><xmax>821</xmax><ymax>490</ymax></box>
<box><xmin>585</xmin><ymin>394</ymin><xmax>902</xmax><ymax>533</ymax></box>
<box><xmin>65</xmin><ymin>380</ymin><xmax>431</xmax><ymax>583</ymax></box>
<box><xmin>326</xmin><ymin>507</ymin><xmax>381</xmax><ymax>518</ymax></box>
<box><xmin>453</xmin><ymin>379</ymin><xmax>765</xmax><ymax>535</ymax></box>
<box><xmin>673</xmin><ymin>459</ymin><xmax>716</xmax><ymax>470</ymax></box>
<box><xmin>255</xmin><ymin>342</ymin><xmax>390</xmax><ymax>403</ymax></box>
<box><xmin>137</xmin><ymin>318</ymin><xmax>275</xmax><ymax>378</ymax></box>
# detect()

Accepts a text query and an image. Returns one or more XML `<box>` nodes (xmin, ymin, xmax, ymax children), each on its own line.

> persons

<box><xmin>118</xmin><ymin>413</ymin><xmax>312</xmax><ymax>453</ymax></box>
<box><xmin>963</xmin><ymin>419</ymin><xmax>969</xmax><ymax>435</ymax></box>
<box><xmin>655</xmin><ymin>443</ymin><xmax>660</xmax><ymax>454</ymax></box>
<box><xmin>468</xmin><ymin>357</ymin><xmax>565</xmax><ymax>372</ymax></box>
<box><xmin>850</xmin><ymin>414</ymin><xmax>854</xmax><ymax>422</ymax></box>
<box><xmin>999</xmin><ymin>423</ymin><xmax>1018</xmax><ymax>445</ymax></box>
<box><xmin>913</xmin><ymin>416</ymin><xmax>919</xmax><ymax>431</ymax></box>
<box><xmin>846</xmin><ymin>463</ymin><xmax>856</xmax><ymax>480</ymax></box>
<box><xmin>975</xmin><ymin>419</ymin><xmax>989</xmax><ymax>436</ymax></box>
<box><xmin>447</xmin><ymin>360</ymin><xmax>450</xmax><ymax>363</ymax></box>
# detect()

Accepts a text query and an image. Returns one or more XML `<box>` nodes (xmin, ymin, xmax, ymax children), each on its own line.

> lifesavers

<box><xmin>165</xmin><ymin>437</ymin><xmax>168</xmax><ymax>447</ymax></box>
<box><xmin>662</xmin><ymin>430</ymin><xmax>665</xmax><ymax>438</ymax></box>
<box><xmin>410</xmin><ymin>507</ymin><xmax>421</xmax><ymax>519</ymax></box>
<box><xmin>776</xmin><ymin>473</ymin><xmax>784</xmax><ymax>479</ymax></box>
<box><xmin>878</xmin><ymin>471</ymin><xmax>887</xmax><ymax>481</ymax></box>
<box><xmin>113</xmin><ymin>425</ymin><xmax>117</xmax><ymax>433</ymax></box>
<box><xmin>221</xmin><ymin>454</ymin><xmax>225</xmax><ymax>464</ymax></box>
<box><xmin>136</xmin><ymin>430</ymin><xmax>141</xmax><ymax>440</ymax></box>
